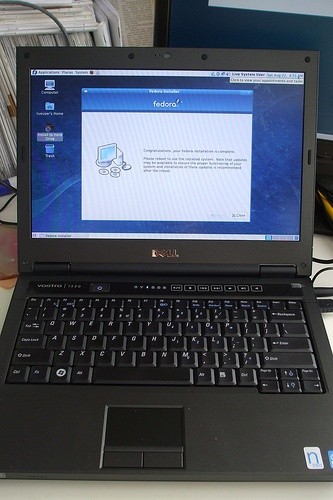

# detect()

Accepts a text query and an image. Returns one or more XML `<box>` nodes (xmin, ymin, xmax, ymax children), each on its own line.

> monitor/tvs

<box><xmin>154</xmin><ymin>0</ymin><xmax>333</xmax><ymax>173</ymax></box>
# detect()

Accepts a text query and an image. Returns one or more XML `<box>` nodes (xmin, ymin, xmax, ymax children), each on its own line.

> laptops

<box><xmin>0</xmin><ymin>47</ymin><xmax>332</xmax><ymax>482</ymax></box>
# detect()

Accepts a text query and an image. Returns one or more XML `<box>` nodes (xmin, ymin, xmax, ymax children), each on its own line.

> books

<box><xmin>1</xmin><ymin>0</ymin><xmax>125</xmax><ymax>183</ymax></box>
